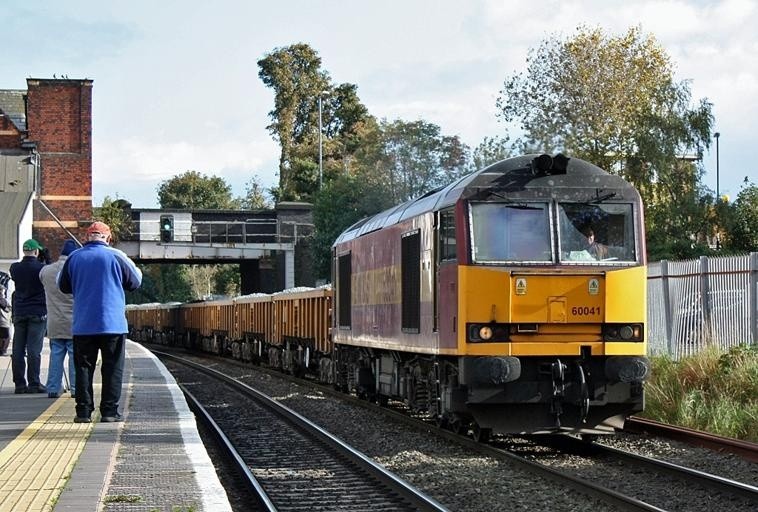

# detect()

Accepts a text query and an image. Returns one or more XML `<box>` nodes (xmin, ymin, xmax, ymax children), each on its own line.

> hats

<box><xmin>23</xmin><ymin>239</ymin><xmax>43</xmax><ymax>250</ymax></box>
<box><xmin>88</xmin><ymin>221</ymin><xmax>111</xmax><ymax>237</ymax></box>
<box><xmin>61</xmin><ymin>239</ymin><xmax>75</xmax><ymax>255</ymax></box>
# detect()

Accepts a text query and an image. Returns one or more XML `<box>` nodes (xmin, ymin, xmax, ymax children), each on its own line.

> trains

<box><xmin>125</xmin><ymin>153</ymin><xmax>651</xmax><ymax>444</ymax></box>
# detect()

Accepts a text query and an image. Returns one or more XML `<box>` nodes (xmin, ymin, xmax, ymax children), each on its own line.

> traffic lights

<box><xmin>160</xmin><ymin>214</ymin><xmax>174</xmax><ymax>243</ymax></box>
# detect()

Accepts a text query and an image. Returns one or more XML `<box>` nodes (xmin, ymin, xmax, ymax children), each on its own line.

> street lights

<box><xmin>318</xmin><ymin>91</ymin><xmax>329</xmax><ymax>192</ymax></box>
<box><xmin>714</xmin><ymin>132</ymin><xmax>720</xmax><ymax>252</ymax></box>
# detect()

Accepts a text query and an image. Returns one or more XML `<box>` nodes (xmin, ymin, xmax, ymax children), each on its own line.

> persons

<box><xmin>9</xmin><ymin>238</ymin><xmax>49</xmax><ymax>394</ymax></box>
<box><xmin>580</xmin><ymin>227</ymin><xmax>609</xmax><ymax>260</ymax></box>
<box><xmin>38</xmin><ymin>239</ymin><xmax>78</xmax><ymax>398</ymax></box>
<box><xmin>57</xmin><ymin>221</ymin><xmax>144</xmax><ymax>424</ymax></box>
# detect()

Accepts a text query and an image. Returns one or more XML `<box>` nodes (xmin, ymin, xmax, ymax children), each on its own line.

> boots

<box><xmin>14</xmin><ymin>384</ymin><xmax>46</xmax><ymax>393</ymax></box>
<box><xmin>1</xmin><ymin>352</ymin><xmax>8</xmax><ymax>356</ymax></box>
<box><xmin>48</xmin><ymin>392</ymin><xmax>75</xmax><ymax>398</ymax></box>
<box><xmin>100</xmin><ymin>414</ymin><xmax>124</xmax><ymax>422</ymax></box>
<box><xmin>74</xmin><ymin>415</ymin><xmax>92</xmax><ymax>423</ymax></box>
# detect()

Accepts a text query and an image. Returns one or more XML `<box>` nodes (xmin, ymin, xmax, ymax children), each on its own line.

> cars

<box><xmin>674</xmin><ymin>289</ymin><xmax>745</xmax><ymax>344</ymax></box>
